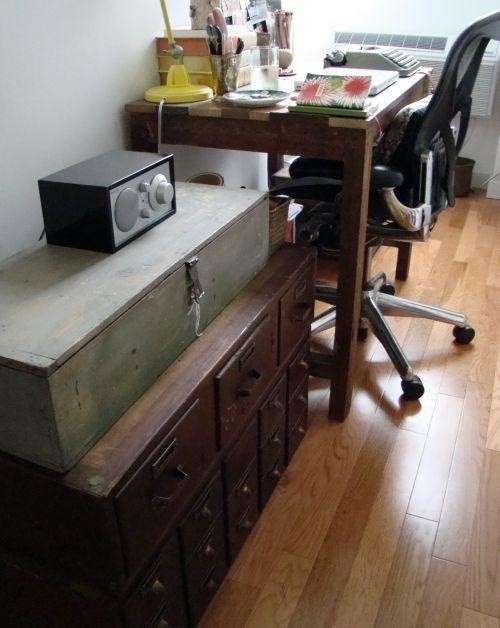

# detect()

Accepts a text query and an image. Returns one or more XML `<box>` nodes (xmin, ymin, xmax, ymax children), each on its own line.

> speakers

<box><xmin>37</xmin><ymin>147</ymin><xmax>177</xmax><ymax>254</ymax></box>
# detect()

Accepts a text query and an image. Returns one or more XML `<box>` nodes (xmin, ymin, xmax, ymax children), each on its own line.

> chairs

<box><xmin>268</xmin><ymin>13</ymin><xmax>500</xmax><ymax>401</ymax></box>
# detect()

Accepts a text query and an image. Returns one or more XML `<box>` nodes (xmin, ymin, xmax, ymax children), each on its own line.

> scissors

<box><xmin>206</xmin><ymin>25</ymin><xmax>222</xmax><ymax>42</ymax></box>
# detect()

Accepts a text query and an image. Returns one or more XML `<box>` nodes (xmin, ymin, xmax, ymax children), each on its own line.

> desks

<box><xmin>124</xmin><ymin>66</ymin><xmax>434</xmax><ymax>425</ymax></box>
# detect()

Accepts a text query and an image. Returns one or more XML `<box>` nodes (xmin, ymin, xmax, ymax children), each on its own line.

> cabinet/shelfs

<box><xmin>0</xmin><ymin>241</ymin><xmax>319</xmax><ymax>628</ymax></box>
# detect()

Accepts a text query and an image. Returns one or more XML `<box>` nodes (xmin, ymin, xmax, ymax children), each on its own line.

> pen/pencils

<box><xmin>208</xmin><ymin>35</ymin><xmax>244</xmax><ymax>56</ymax></box>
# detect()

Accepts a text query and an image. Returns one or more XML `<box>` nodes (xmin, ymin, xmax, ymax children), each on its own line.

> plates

<box><xmin>222</xmin><ymin>90</ymin><xmax>291</xmax><ymax>109</ymax></box>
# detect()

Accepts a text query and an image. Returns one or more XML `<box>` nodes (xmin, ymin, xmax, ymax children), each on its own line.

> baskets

<box><xmin>268</xmin><ymin>196</ymin><xmax>291</xmax><ymax>257</ymax></box>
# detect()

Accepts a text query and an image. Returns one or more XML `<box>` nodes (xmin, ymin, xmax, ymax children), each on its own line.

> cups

<box><xmin>267</xmin><ymin>11</ymin><xmax>297</xmax><ymax>76</ymax></box>
<box><xmin>250</xmin><ymin>46</ymin><xmax>279</xmax><ymax>91</ymax></box>
<box><xmin>208</xmin><ymin>53</ymin><xmax>241</xmax><ymax>101</ymax></box>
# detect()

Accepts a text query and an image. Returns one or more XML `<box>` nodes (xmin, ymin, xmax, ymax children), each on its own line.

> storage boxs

<box><xmin>0</xmin><ymin>181</ymin><xmax>271</xmax><ymax>473</ymax></box>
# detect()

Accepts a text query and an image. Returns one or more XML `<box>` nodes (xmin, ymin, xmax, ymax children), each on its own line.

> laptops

<box><xmin>294</xmin><ymin>67</ymin><xmax>400</xmax><ymax>96</ymax></box>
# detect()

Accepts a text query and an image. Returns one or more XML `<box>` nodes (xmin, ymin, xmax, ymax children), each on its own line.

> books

<box><xmin>288</xmin><ymin>101</ymin><xmax>380</xmax><ymax>117</ymax></box>
<box><xmin>316</xmin><ymin>66</ymin><xmax>401</xmax><ymax>98</ymax></box>
<box><xmin>297</xmin><ymin>72</ymin><xmax>373</xmax><ymax>109</ymax></box>
